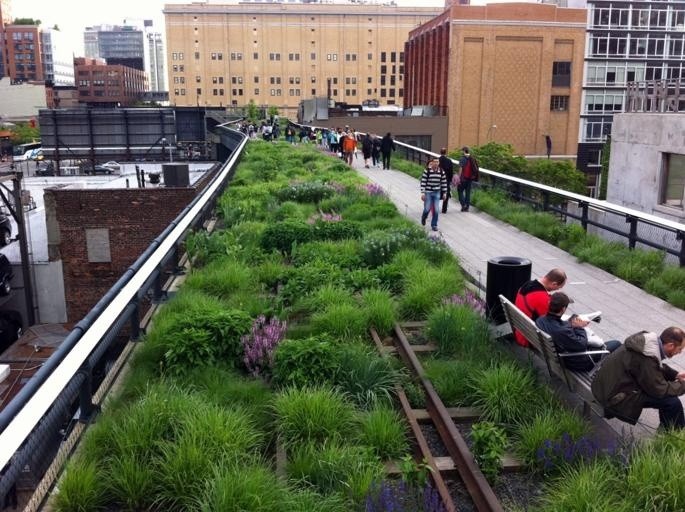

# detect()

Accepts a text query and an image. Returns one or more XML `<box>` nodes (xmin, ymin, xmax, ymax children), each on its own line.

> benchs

<box><xmin>495</xmin><ymin>296</ymin><xmax>613</xmax><ymax>392</ymax></box>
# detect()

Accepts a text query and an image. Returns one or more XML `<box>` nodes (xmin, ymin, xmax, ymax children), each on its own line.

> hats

<box><xmin>551</xmin><ymin>292</ymin><xmax>574</xmax><ymax>305</ymax></box>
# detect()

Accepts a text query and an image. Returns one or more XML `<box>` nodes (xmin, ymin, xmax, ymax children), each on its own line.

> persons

<box><xmin>419</xmin><ymin>156</ymin><xmax>448</xmax><ymax>232</ymax></box>
<box><xmin>534</xmin><ymin>291</ymin><xmax>623</xmax><ymax>372</ymax></box>
<box><xmin>591</xmin><ymin>327</ymin><xmax>685</xmax><ymax>433</ymax></box>
<box><xmin>456</xmin><ymin>147</ymin><xmax>480</xmax><ymax>212</ymax></box>
<box><xmin>514</xmin><ymin>268</ymin><xmax>568</xmax><ymax>348</ymax></box>
<box><xmin>421</xmin><ymin>161</ymin><xmax>440</xmax><ymax>220</ymax></box>
<box><xmin>235</xmin><ymin>112</ymin><xmax>397</xmax><ymax>172</ymax></box>
<box><xmin>437</xmin><ymin>148</ymin><xmax>453</xmax><ymax>213</ymax></box>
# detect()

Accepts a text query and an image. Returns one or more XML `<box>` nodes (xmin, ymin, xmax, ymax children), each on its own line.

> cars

<box><xmin>34</xmin><ymin>159</ymin><xmax>121</xmax><ymax>174</ymax></box>
<box><xmin>0</xmin><ymin>212</ymin><xmax>29</xmax><ymax>356</ymax></box>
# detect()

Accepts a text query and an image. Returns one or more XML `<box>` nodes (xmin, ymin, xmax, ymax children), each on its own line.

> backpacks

<box><xmin>463</xmin><ymin>156</ymin><xmax>477</xmax><ymax>180</ymax></box>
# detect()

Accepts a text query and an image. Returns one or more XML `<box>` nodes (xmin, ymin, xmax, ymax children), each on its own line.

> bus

<box><xmin>11</xmin><ymin>143</ymin><xmax>42</xmax><ymax>161</ymax></box>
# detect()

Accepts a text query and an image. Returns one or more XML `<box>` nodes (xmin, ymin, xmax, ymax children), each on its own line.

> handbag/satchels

<box><xmin>583</xmin><ymin>326</ymin><xmax>603</xmax><ymax>347</ymax></box>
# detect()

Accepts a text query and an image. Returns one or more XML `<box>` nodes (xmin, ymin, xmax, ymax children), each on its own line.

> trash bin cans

<box><xmin>487</xmin><ymin>257</ymin><xmax>531</xmax><ymax>322</ymax></box>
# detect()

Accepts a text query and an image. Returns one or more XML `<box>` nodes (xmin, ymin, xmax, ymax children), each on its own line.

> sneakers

<box><xmin>432</xmin><ymin>226</ymin><xmax>437</xmax><ymax>231</ymax></box>
<box><xmin>422</xmin><ymin>216</ymin><xmax>425</xmax><ymax>225</ymax></box>
<box><xmin>461</xmin><ymin>203</ymin><xmax>469</xmax><ymax>212</ymax></box>
